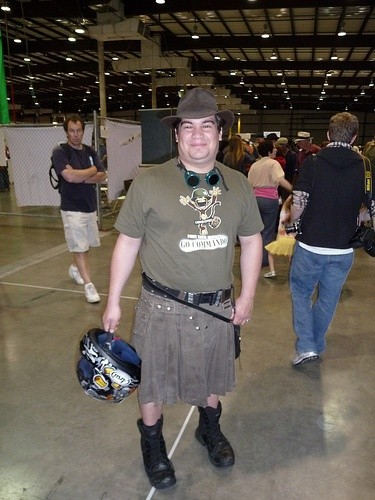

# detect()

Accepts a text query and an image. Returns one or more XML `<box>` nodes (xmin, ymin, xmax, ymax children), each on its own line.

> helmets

<box><xmin>77</xmin><ymin>328</ymin><xmax>142</xmax><ymax>403</ymax></box>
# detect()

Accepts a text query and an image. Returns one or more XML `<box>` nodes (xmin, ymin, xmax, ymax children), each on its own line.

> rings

<box><xmin>246</xmin><ymin>320</ymin><xmax>249</xmax><ymax>321</ymax></box>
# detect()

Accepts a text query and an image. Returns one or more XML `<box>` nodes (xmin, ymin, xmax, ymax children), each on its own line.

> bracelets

<box><xmin>82</xmin><ymin>180</ymin><xmax>85</xmax><ymax>182</ymax></box>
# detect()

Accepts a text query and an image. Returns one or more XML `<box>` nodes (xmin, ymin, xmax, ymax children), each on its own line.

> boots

<box><xmin>195</xmin><ymin>401</ymin><xmax>235</xmax><ymax>468</ymax></box>
<box><xmin>137</xmin><ymin>415</ymin><xmax>176</xmax><ymax>488</ymax></box>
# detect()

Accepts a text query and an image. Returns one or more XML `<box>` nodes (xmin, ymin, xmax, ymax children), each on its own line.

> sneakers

<box><xmin>85</xmin><ymin>283</ymin><xmax>101</xmax><ymax>303</ymax></box>
<box><xmin>68</xmin><ymin>264</ymin><xmax>84</xmax><ymax>285</ymax></box>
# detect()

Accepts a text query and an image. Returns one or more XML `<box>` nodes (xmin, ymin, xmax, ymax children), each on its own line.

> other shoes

<box><xmin>264</xmin><ymin>271</ymin><xmax>277</xmax><ymax>279</ymax></box>
<box><xmin>293</xmin><ymin>351</ymin><xmax>319</xmax><ymax>366</ymax></box>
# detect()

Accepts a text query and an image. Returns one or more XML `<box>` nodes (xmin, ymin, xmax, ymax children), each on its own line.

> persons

<box><xmin>102</xmin><ymin>87</ymin><xmax>264</xmax><ymax>488</ymax></box>
<box><xmin>215</xmin><ymin>133</ymin><xmax>375</xmax><ymax>278</ymax></box>
<box><xmin>51</xmin><ymin>114</ymin><xmax>107</xmax><ymax>303</ymax></box>
<box><xmin>288</xmin><ymin>111</ymin><xmax>366</xmax><ymax>365</ymax></box>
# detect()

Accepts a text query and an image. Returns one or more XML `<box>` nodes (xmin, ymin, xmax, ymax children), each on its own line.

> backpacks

<box><xmin>48</xmin><ymin>143</ymin><xmax>93</xmax><ymax>194</ymax></box>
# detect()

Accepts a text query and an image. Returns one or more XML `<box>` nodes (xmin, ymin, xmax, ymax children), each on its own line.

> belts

<box><xmin>142</xmin><ymin>277</ymin><xmax>231</xmax><ymax>306</ymax></box>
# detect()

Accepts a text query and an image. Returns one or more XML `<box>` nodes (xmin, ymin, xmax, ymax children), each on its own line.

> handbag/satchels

<box><xmin>234</xmin><ymin>325</ymin><xmax>241</xmax><ymax>359</ymax></box>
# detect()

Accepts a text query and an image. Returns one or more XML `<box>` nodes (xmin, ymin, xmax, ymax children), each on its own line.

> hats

<box><xmin>161</xmin><ymin>88</ymin><xmax>235</xmax><ymax>130</ymax></box>
<box><xmin>294</xmin><ymin>131</ymin><xmax>310</xmax><ymax>141</ymax></box>
